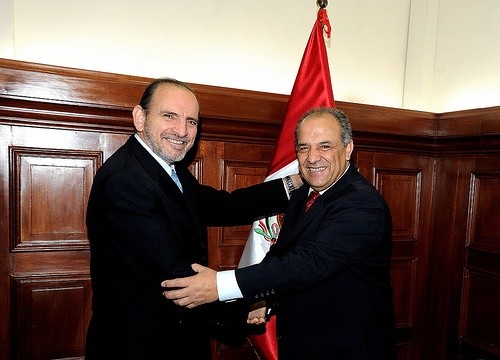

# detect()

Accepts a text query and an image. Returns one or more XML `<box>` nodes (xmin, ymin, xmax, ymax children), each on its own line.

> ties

<box><xmin>171</xmin><ymin>169</ymin><xmax>184</xmax><ymax>195</ymax></box>
<box><xmin>304</xmin><ymin>192</ymin><xmax>321</xmax><ymax>214</ymax></box>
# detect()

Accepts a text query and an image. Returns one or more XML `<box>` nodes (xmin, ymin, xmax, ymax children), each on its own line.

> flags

<box><xmin>227</xmin><ymin>10</ymin><xmax>339</xmax><ymax>360</ymax></box>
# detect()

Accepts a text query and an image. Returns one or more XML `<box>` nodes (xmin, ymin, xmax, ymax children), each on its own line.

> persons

<box><xmin>81</xmin><ymin>76</ymin><xmax>313</xmax><ymax>360</ymax></box>
<box><xmin>159</xmin><ymin>105</ymin><xmax>396</xmax><ymax>360</ymax></box>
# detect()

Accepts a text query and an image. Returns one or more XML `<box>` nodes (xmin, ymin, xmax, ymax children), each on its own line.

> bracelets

<box><xmin>284</xmin><ymin>175</ymin><xmax>295</xmax><ymax>196</ymax></box>
